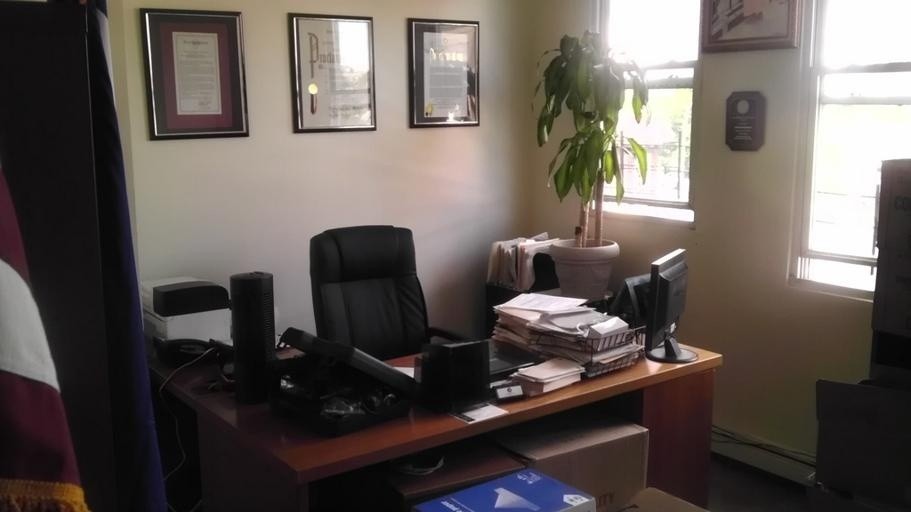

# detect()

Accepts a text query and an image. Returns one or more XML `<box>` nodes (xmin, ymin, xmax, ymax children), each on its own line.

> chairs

<box><xmin>309</xmin><ymin>225</ymin><xmax>462</xmax><ymax>361</ymax></box>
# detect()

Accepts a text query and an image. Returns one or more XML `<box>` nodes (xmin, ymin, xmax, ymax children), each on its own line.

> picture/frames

<box><xmin>140</xmin><ymin>8</ymin><xmax>249</xmax><ymax>140</ymax></box>
<box><xmin>407</xmin><ymin>18</ymin><xmax>480</xmax><ymax>128</ymax></box>
<box><xmin>287</xmin><ymin>12</ymin><xmax>377</xmax><ymax>134</ymax></box>
<box><xmin>700</xmin><ymin>0</ymin><xmax>803</xmax><ymax>54</ymax></box>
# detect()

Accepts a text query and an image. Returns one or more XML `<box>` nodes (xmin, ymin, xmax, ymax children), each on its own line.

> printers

<box><xmin>141</xmin><ymin>275</ymin><xmax>233</xmax><ymax>347</ymax></box>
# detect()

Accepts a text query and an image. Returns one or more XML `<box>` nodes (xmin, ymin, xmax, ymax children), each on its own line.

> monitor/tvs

<box><xmin>643</xmin><ymin>247</ymin><xmax>698</xmax><ymax>365</ymax></box>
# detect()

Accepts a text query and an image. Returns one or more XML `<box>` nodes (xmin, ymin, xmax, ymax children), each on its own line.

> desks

<box><xmin>147</xmin><ymin>338</ymin><xmax>724</xmax><ymax>512</ymax></box>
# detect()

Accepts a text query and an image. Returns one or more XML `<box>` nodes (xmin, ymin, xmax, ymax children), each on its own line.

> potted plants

<box><xmin>531</xmin><ymin>29</ymin><xmax>648</xmax><ymax>301</ymax></box>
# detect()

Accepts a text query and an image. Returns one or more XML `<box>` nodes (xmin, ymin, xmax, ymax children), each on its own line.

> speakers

<box><xmin>231</xmin><ymin>272</ymin><xmax>278</xmax><ymax>403</ymax></box>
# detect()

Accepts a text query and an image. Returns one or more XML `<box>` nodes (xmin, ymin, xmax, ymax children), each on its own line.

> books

<box><xmin>486</xmin><ymin>232</ymin><xmax>561</xmax><ymax>291</ymax></box>
<box><xmin>492</xmin><ymin>291</ymin><xmax>643</xmax><ymax>377</ymax></box>
<box><xmin>507</xmin><ymin>358</ymin><xmax>585</xmax><ymax>396</ymax></box>
